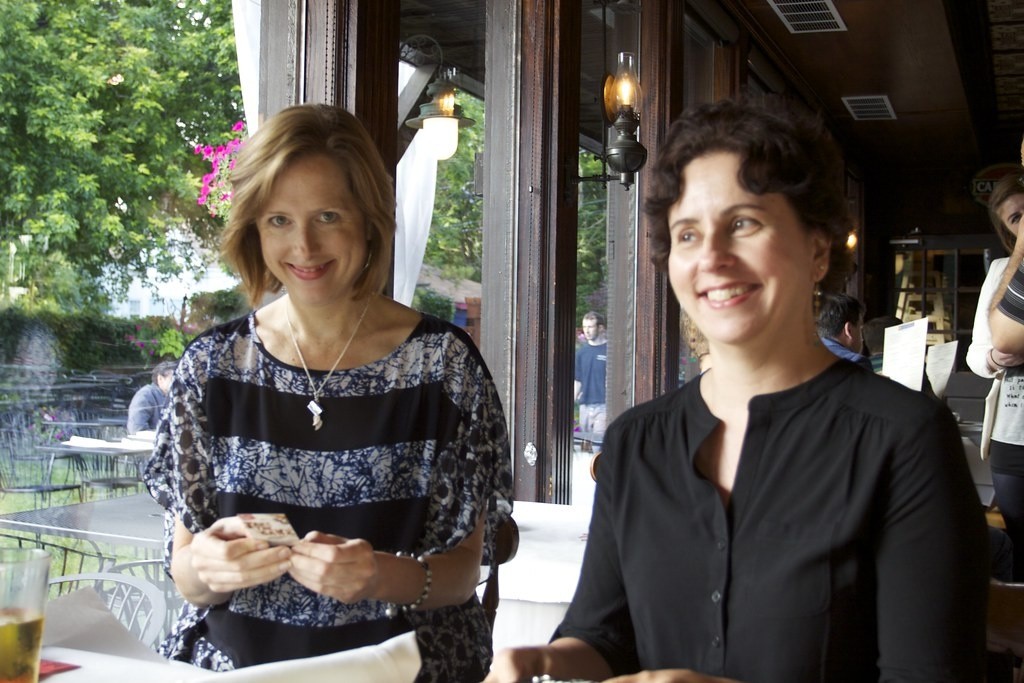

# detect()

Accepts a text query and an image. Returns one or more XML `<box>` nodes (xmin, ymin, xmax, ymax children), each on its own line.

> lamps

<box><xmin>399</xmin><ymin>34</ymin><xmax>477</xmax><ymax>161</ymax></box>
<box><xmin>578</xmin><ymin>52</ymin><xmax>648</xmax><ymax>191</ymax></box>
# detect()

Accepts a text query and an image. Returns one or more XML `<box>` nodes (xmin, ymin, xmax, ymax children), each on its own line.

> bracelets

<box><xmin>385</xmin><ymin>551</ymin><xmax>433</xmax><ymax>617</ymax></box>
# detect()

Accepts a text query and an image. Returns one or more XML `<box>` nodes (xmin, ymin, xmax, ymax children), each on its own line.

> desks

<box><xmin>34</xmin><ymin>442</ymin><xmax>154</xmax><ymax>503</ymax></box>
<box><xmin>40</xmin><ymin>420</ymin><xmax>106</xmax><ymax>444</ymax></box>
<box><xmin>0</xmin><ymin>492</ymin><xmax>164</xmax><ymax>592</ymax></box>
<box><xmin>99</xmin><ymin>416</ymin><xmax>128</xmax><ymax>438</ymax></box>
<box><xmin>475</xmin><ymin>501</ymin><xmax>591</xmax><ymax>650</ymax></box>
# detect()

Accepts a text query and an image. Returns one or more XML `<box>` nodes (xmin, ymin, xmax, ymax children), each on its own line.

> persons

<box><xmin>482</xmin><ymin>93</ymin><xmax>1024</xmax><ymax>683</ymax></box>
<box><xmin>141</xmin><ymin>104</ymin><xmax>514</xmax><ymax>683</ymax></box>
<box><xmin>574</xmin><ymin>311</ymin><xmax>606</xmax><ymax>435</ymax></box>
<box><xmin>127</xmin><ymin>360</ymin><xmax>178</xmax><ymax>434</ymax></box>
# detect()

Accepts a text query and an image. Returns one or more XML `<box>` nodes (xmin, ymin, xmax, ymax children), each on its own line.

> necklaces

<box><xmin>283</xmin><ymin>297</ymin><xmax>370</xmax><ymax>431</ymax></box>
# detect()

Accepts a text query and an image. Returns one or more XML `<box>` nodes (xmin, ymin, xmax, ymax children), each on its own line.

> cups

<box><xmin>104</xmin><ymin>425</ymin><xmax>127</xmax><ymax>442</ymax></box>
<box><xmin>0</xmin><ymin>548</ymin><xmax>52</xmax><ymax>682</ymax></box>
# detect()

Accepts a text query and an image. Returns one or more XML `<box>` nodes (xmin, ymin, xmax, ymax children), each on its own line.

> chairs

<box><xmin>0</xmin><ymin>367</ymin><xmax>185</xmax><ymax>655</ymax></box>
